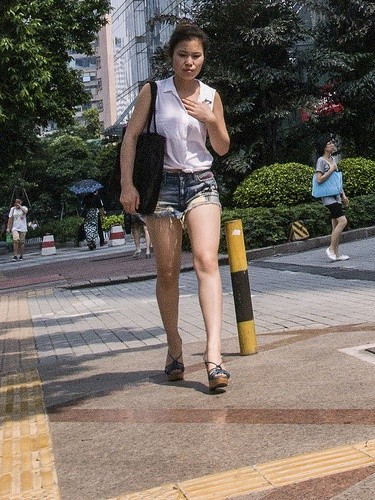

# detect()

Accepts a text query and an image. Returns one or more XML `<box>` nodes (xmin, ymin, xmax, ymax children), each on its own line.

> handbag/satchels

<box><xmin>105</xmin><ymin>79</ymin><xmax>166</xmax><ymax>216</ymax></box>
<box><xmin>311</xmin><ymin>157</ymin><xmax>343</xmax><ymax>198</ymax></box>
<box><xmin>6</xmin><ymin>233</ymin><xmax>13</xmax><ymax>246</ymax></box>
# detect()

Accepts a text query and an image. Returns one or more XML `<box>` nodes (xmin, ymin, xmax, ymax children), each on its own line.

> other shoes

<box><xmin>88</xmin><ymin>241</ymin><xmax>97</xmax><ymax>250</ymax></box>
<box><xmin>11</xmin><ymin>256</ymin><xmax>24</xmax><ymax>261</ymax></box>
<box><xmin>74</xmin><ymin>239</ymin><xmax>79</xmax><ymax>247</ymax></box>
<box><xmin>132</xmin><ymin>249</ymin><xmax>141</xmax><ymax>258</ymax></box>
<box><xmin>146</xmin><ymin>253</ymin><xmax>151</xmax><ymax>259</ymax></box>
<box><xmin>326</xmin><ymin>247</ymin><xmax>350</xmax><ymax>261</ymax></box>
<box><xmin>100</xmin><ymin>241</ymin><xmax>108</xmax><ymax>247</ymax></box>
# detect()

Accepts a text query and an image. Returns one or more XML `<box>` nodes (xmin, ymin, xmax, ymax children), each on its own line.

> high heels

<box><xmin>203</xmin><ymin>360</ymin><xmax>231</xmax><ymax>391</ymax></box>
<box><xmin>164</xmin><ymin>350</ymin><xmax>186</xmax><ymax>382</ymax></box>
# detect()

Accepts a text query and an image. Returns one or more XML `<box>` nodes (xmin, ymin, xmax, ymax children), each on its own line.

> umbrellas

<box><xmin>70</xmin><ymin>178</ymin><xmax>103</xmax><ymax>195</ymax></box>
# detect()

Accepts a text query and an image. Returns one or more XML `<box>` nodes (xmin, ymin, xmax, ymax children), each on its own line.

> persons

<box><xmin>74</xmin><ymin>193</ymin><xmax>107</xmax><ymax>250</ymax></box>
<box><xmin>120</xmin><ymin>24</ymin><xmax>230</xmax><ymax>389</ymax></box>
<box><xmin>312</xmin><ymin>134</ymin><xmax>349</xmax><ymax>261</ymax></box>
<box><xmin>6</xmin><ymin>194</ymin><xmax>28</xmax><ymax>261</ymax></box>
<box><xmin>131</xmin><ymin>215</ymin><xmax>152</xmax><ymax>259</ymax></box>
